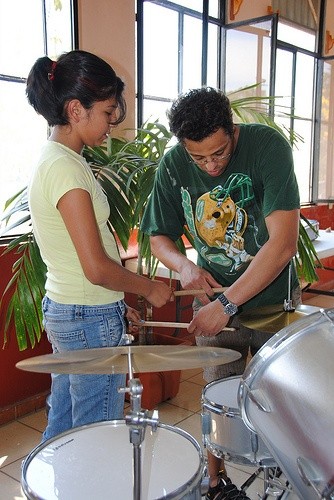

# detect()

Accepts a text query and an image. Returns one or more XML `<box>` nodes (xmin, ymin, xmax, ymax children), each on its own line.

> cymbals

<box><xmin>15</xmin><ymin>345</ymin><xmax>242</xmax><ymax>376</ymax></box>
<box><xmin>235</xmin><ymin>303</ymin><xmax>324</xmax><ymax>335</ymax></box>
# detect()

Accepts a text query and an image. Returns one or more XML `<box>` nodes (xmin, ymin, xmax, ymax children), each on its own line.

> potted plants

<box><xmin>0</xmin><ymin>79</ymin><xmax>324</xmax><ymax>409</ymax></box>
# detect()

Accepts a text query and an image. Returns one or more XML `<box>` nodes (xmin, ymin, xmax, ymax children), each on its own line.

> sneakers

<box><xmin>206</xmin><ymin>478</ymin><xmax>250</xmax><ymax>500</ymax></box>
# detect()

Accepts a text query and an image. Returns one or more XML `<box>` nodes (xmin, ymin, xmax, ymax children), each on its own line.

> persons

<box><xmin>24</xmin><ymin>49</ymin><xmax>173</xmax><ymax>443</ymax></box>
<box><xmin>139</xmin><ymin>86</ymin><xmax>301</xmax><ymax>500</ymax></box>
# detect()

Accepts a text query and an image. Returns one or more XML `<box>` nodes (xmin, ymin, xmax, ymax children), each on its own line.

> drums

<box><xmin>238</xmin><ymin>308</ymin><xmax>334</xmax><ymax>500</ymax></box>
<box><xmin>198</xmin><ymin>373</ymin><xmax>281</xmax><ymax>468</ymax></box>
<box><xmin>20</xmin><ymin>416</ymin><xmax>207</xmax><ymax>500</ymax></box>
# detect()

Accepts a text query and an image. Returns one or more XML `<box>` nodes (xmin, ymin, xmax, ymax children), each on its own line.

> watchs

<box><xmin>217</xmin><ymin>294</ymin><xmax>239</xmax><ymax>316</ymax></box>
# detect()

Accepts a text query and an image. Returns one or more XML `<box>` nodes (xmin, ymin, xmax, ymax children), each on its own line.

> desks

<box><xmin>124</xmin><ymin>229</ymin><xmax>334</xmax><ymax>346</ymax></box>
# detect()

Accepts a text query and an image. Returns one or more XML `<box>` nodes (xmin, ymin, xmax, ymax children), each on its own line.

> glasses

<box><xmin>188</xmin><ymin>133</ymin><xmax>232</xmax><ymax>165</ymax></box>
